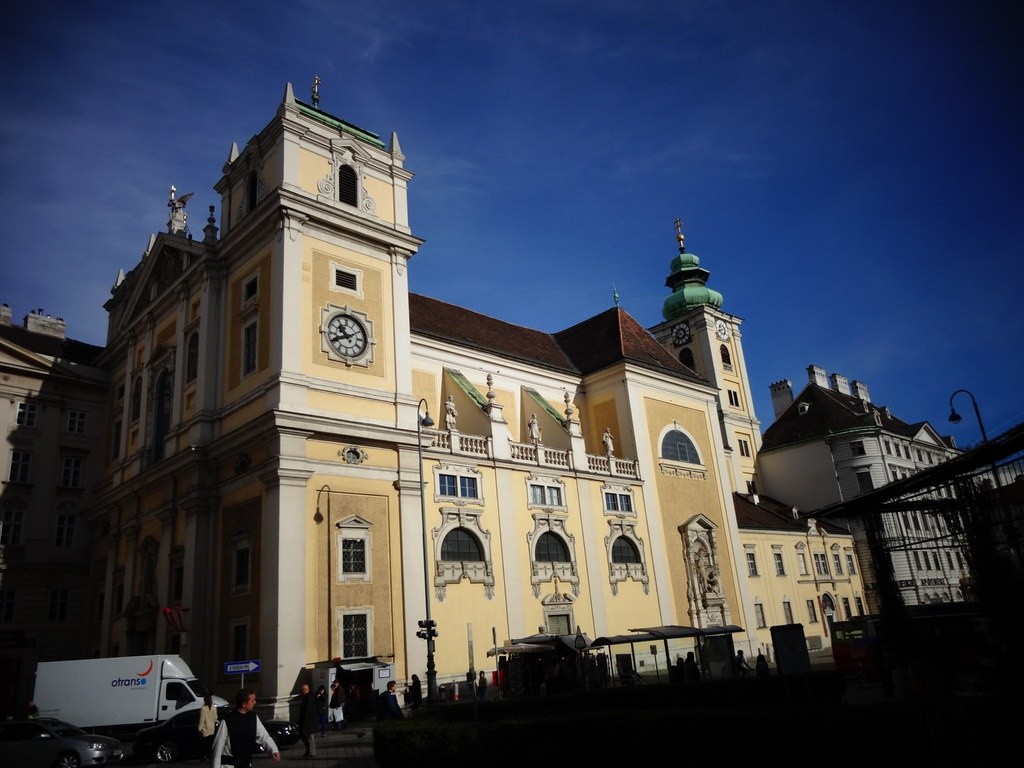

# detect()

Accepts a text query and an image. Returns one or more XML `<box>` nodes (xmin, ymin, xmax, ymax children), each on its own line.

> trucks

<box><xmin>33</xmin><ymin>654</ymin><xmax>230</xmax><ymax>746</ymax></box>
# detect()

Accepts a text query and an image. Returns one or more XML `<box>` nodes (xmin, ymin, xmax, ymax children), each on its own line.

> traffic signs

<box><xmin>223</xmin><ymin>660</ymin><xmax>261</xmax><ymax>674</ymax></box>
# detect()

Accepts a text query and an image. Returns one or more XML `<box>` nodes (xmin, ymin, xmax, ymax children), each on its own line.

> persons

<box><xmin>603</xmin><ymin>428</ymin><xmax>614</xmax><ymax>456</ymax></box>
<box><xmin>478</xmin><ymin>671</ymin><xmax>488</xmax><ymax>702</ymax></box>
<box><xmin>684</xmin><ymin>652</ymin><xmax>701</xmax><ymax>680</ymax></box>
<box><xmin>197</xmin><ymin>696</ymin><xmax>218</xmax><ymax>760</ymax></box>
<box><xmin>375</xmin><ymin>681</ymin><xmax>403</xmax><ymax>721</ymax></box>
<box><xmin>404</xmin><ymin>674</ymin><xmax>422</xmax><ymax>703</ymax></box>
<box><xmin>298</xmin><ymin>683</ymin><xmax>316</xmax><ymax>758</ymax></box>
<box><xmin>530</xmin><ymin>413</ymin><xmax>540</xmax><ymax>443</ymax></box>
<box><xmin>211</xmin><ymin>688</ymin><xmax>281</xmax><ymax>768</ymax></box>
<box><xmin>315</xmin><ymin>679</ymin><xmax>345</xmax><ymax>738</ymax></box>
<box><xmin>735</xmin><ymin>650</ymin><xmax>752</xmax><ymax>682</ymax></box>
<box><xmin>444</xmin><ymin>396</ymin><xmax>458</xmax><ymax>429</ymax></box>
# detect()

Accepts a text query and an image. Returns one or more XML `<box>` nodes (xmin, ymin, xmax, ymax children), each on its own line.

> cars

<box><xmin>0</xmin><ymin>716</ymin><xmax>124</xmax><ymax>768</ymax></box>
<box><xmin>132</xmin><ymin>707</ymin><xmax>300</xmax><ymax>765</ymax></box>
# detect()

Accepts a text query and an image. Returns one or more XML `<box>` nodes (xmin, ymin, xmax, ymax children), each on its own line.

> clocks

<box><xmin>325</xmin><ymin>312</ymin><xmax>370</xmax><ymax>361</ymax></box>
<box><xmin>672</xmin><ymin>323</ymin><xmax>689</xmax><ymax>345</ymax></box>
<box><xmin>716</xmin><ymin>320</ymin><xmax>728</xmax><ymax>339</ymax></box>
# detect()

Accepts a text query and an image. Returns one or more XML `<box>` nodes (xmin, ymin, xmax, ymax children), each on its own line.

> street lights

<box><xmin>416</xmin><ymin>397</ymin><xmax>439</xmax><ymax>710</ymax></box>
<box><xmin>948</xmin><ymin>389</ymin><xmax>1024</xmax><ymax>565</ymax></box>
